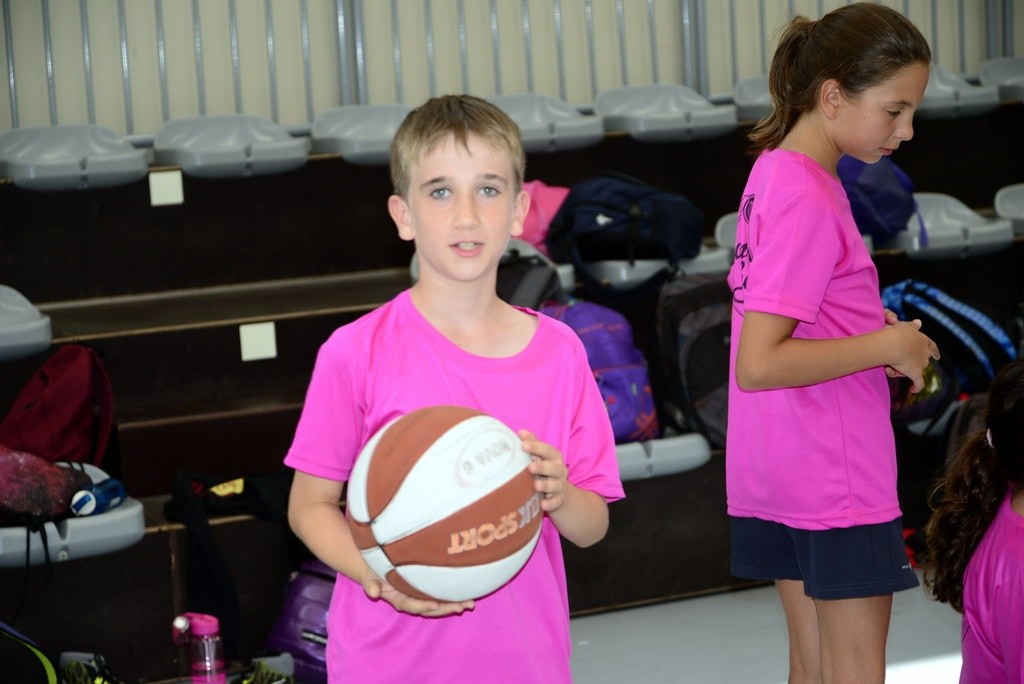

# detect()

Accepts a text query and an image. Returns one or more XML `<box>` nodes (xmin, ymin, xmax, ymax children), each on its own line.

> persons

<box><xmin>286</xmin><ymin>95</ymin><xmax>622</xmax><ymax>683</ymax></box>
<box><xmin>726</xmin><ymin>1</ymin><xmax>942</xmax><ymax>684</ymax></box>
<box><xmin>927</xmin><ymin>364</ymin><xmax>1024</xmax><ymax>684</ymax></box>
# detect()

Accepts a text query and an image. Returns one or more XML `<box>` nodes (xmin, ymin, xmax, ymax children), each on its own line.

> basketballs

<box><xmin>347</xmin><ymin>404</ymin><xmax>545</xmax><ymax>604</ymax></box>
